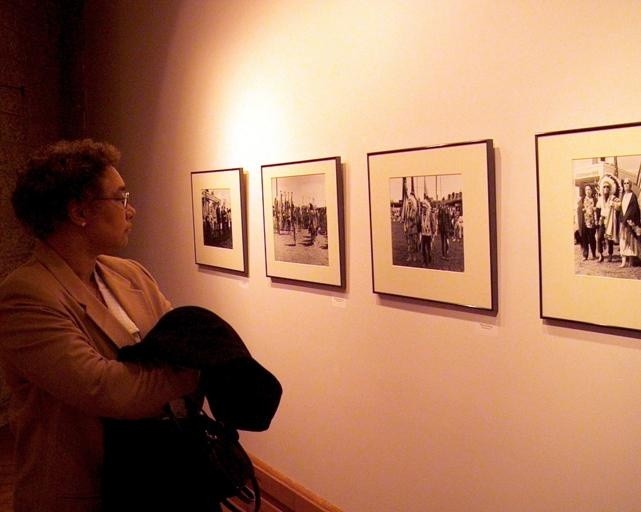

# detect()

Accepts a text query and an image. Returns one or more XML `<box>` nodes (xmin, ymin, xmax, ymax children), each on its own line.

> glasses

<box><xmin>93</xmin><ymin>193</ymin><xmax>129</xmax><ymax>207</ymax></box>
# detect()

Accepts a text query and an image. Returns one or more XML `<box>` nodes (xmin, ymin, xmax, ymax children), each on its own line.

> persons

<box><xmin>577</xmin><ymin>174</ymin><xmax>641</xmax><ymax>268</ymax></box>
<box><xmin>403</xmin><ymin>192</ymin><xmax>463</xmax><ymax>266</ymax></box>
<box><xmin>216</xmin><ymin>201</ymin><xmax>231</xmax><ymax>242</ymax></box>
<box><xmin>274</xmin><ymin>198</ymin><xmax>321</xmax><ymax>246</ymax></box>
<box><xmin>0</xmin><ymin>137</ymin><xmax>205</xmax><ymax>512</ymax></box>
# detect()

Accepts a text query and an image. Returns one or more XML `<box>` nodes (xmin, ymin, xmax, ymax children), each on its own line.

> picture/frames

<box><xmin>366</xmin><ymin>136</ymin><xmax>496</xmax><ymax>314</ymax></box>
<box><xmin>260</xmin><ymin>155</ymin><xmax>344</xmax><ymax>289</ymax></box>
<box><xmin>190</xmin><ymin>166</ymin><xmax>249</xmax><ymax>278</ymax></box>
<box><xmin>533</xmin><ymin>122</ymin><xmax>640</xmax><ymax>333</ymax></box>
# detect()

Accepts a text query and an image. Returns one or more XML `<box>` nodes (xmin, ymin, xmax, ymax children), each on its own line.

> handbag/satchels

<box><xmin>104</xmin><ymin>406</ymin><xmax>261</xmax><ymax>512</ymax></box>
